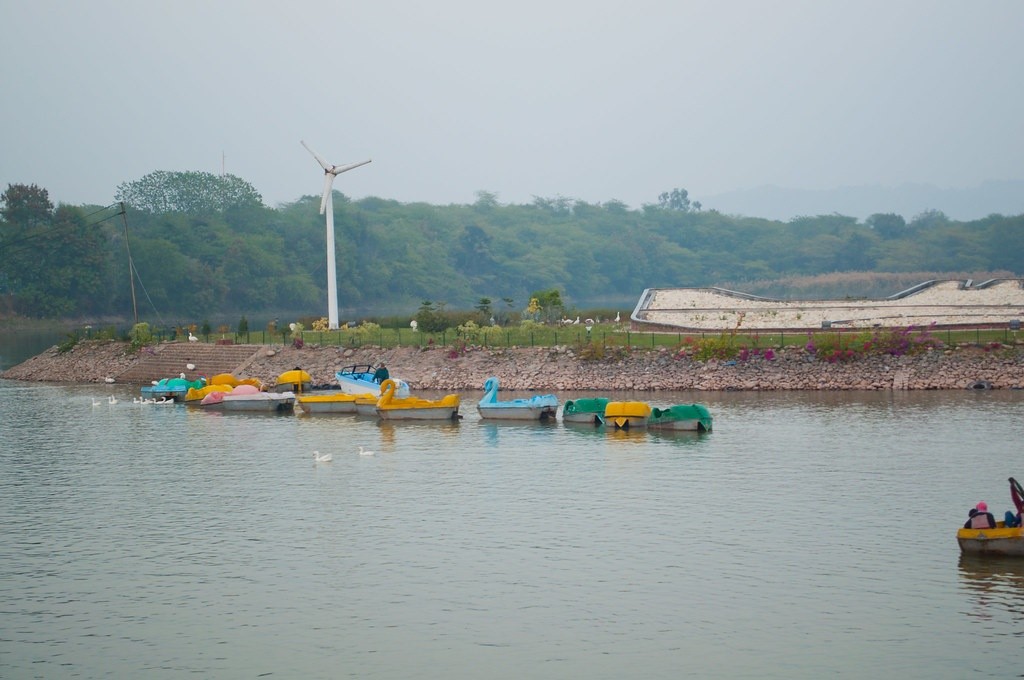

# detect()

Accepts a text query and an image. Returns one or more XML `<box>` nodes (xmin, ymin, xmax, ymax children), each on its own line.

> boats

<box><xmin>563</xmin><ymin>396</ymin><xmax>610</xmax><ymax>425</ymax></box>
<box><xmin>142</xmin><ymin>362</ymin><xmax>464</xmax><ymax>424</ymax></box>
<box><xmin>476</xmin><ymin>377</ymin><xmax>559</xmax><ymax>421</ymax></box>
<box><xmin>647</xmin><ymin>405</ymin><xmax>714</xmax><ymax>432</ymax></box>
<box><xmin>955</xmin><ymin>476</ymin><xmax>1024</xmax><ymax>557</ymax></box>
<box><xmin>603</xmin><ymin>401</ymin><xmax>653</xmax><ymax>428</ymax></box>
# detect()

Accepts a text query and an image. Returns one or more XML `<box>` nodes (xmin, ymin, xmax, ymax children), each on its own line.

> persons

<box><xmin>372</xmin><ymin>362</ymin><xmax>389</xmax><ymax>385</ymax></box>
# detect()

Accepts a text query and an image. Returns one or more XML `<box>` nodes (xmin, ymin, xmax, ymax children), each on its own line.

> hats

<box><xmin>969</xmin><ymin>508</ymin><xmax>979</xmax><ymax>517</ymax></box>
